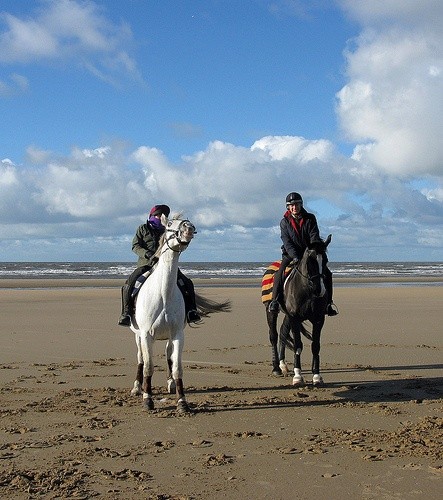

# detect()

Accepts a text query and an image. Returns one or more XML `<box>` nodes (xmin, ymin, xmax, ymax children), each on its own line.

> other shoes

<box><xmin>270</xmin><ymin>304</ymin><xmax>278</xmax><ymax>311</ymax></box>
<box><xmin>186</xmin><ymin>305</ymin><xmax>200</xmax><ymax>323</ymax></box>
<box><xmin>119</xmin><ymin>305</ymin><xmax>133</xmax><ymax>324</ymax></box>
<box><xmin>328</xmin><ymin>309</ymin><xmax>336</xmax><ymax>316</ymax></box>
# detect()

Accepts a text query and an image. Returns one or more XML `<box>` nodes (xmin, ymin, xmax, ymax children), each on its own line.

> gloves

<box><xmin>146</xmin><ymin>252</ymin><xmax>156</xmax><ymax>260</ymax></box>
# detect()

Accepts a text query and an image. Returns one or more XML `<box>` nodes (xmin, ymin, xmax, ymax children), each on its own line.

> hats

<box><xmin>149</xmin><ymin>205</ymin><xmax>170</xmax><ymax>218</ymax></box>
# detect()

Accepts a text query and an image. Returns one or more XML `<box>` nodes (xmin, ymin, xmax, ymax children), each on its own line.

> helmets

<box><xmin>286</xmin><ymin>193</ymin><xmax>302</xmax><ymax>205</ymax></box>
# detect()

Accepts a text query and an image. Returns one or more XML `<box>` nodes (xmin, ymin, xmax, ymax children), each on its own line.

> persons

<box><xmin>270</xmin><ymin>192</ymin><xmax>337</xmax><ymax>316</ymax></box>
<box><xmin>118</xmin><ymin>204</ymin><xmax>201</xmax><ymax>327</ymax></box>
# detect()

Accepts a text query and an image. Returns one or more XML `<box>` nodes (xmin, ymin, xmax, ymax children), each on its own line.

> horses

<box><xmin>261</xmin><ymin>233</ymin><xmax>332</xmax><ymax>386</ymax></box>
<box><xmin>128</xmin><ymin>210</ymin><xmax>232</xmax><ymax>416</ymax></box>
